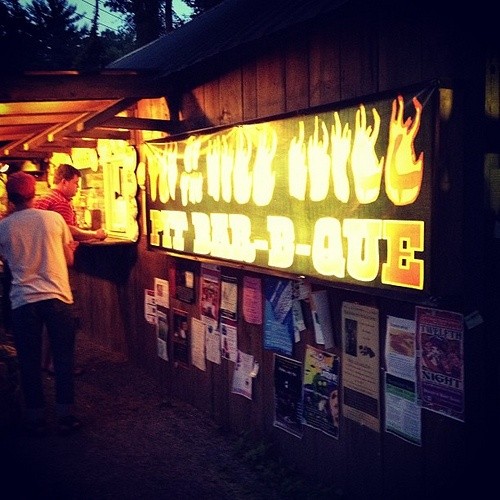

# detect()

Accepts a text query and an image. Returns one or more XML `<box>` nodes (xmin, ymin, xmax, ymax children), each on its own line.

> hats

<box><xmin>3</xmin><ymin>172</ymin><xmax>36</xmax><ymax>199</ymax></box>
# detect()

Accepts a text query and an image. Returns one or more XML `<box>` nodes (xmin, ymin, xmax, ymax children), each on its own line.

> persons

<box><xmin>0</xmin><ymin>170</ymin><xmax>84</xmax><ymax>429</ymax></box>
<box><xmin>33</xmin><ymin>164</ymin><xmax>109</xmax><ymax>241</ymax></box>
